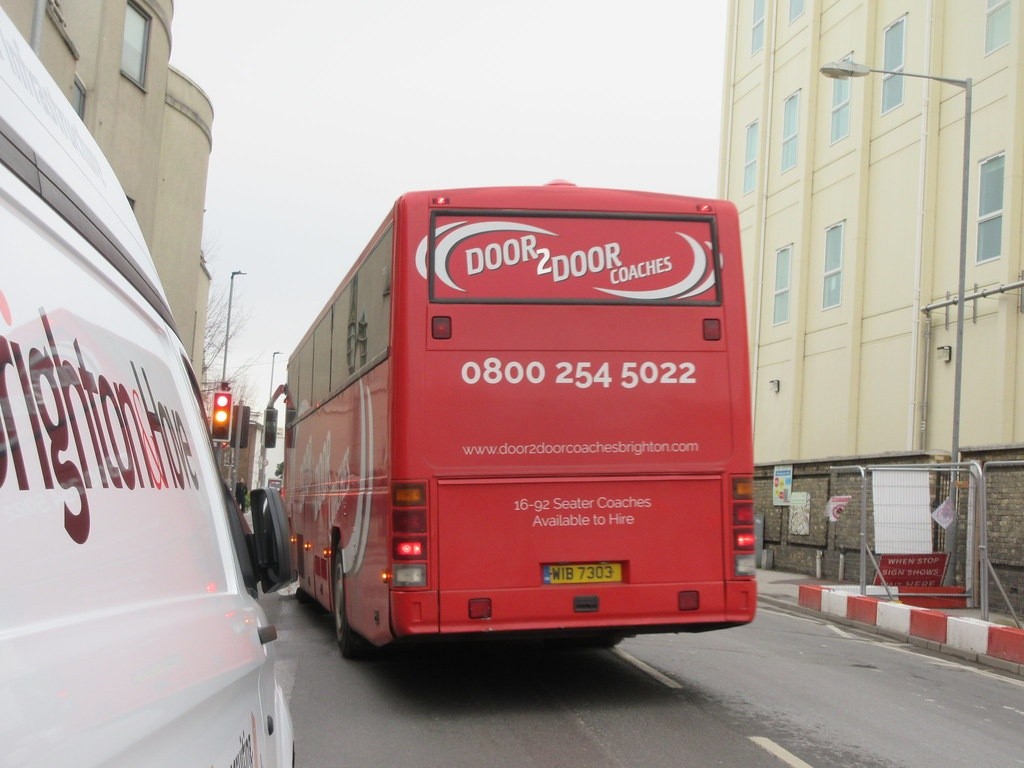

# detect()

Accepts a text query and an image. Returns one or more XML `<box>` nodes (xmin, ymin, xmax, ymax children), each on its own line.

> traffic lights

<box><xmin>210</xmin><ymin>391</ymin><xmax>234</xmax><ymax>442</ymax></box>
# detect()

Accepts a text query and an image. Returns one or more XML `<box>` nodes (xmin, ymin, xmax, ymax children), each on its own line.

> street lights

<box><xmin>214</xmin><ymin>271</ymin><xmax>249</xmax><ymax>471</ymax></box>
<box><xmin>260</xmin><ymin>351</ymin><xmax>284</xmax><ymax>487</ymax></box>
<box><xmin>817</xmin><ymin>58</ymin><xmax>973</xmax><ymax>588</ymax></box>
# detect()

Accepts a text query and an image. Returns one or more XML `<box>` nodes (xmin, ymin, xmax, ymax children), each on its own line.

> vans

<box><xmin>0</xmin><ymin>6</ymin><xmax>298</xmax><ymax>767</ymax></box>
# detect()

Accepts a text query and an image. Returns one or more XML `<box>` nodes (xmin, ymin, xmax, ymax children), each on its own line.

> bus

<box><xmin>264</xmin><ymin>177</ymin><xmax>758</xmax><ymax>663</ymax></box>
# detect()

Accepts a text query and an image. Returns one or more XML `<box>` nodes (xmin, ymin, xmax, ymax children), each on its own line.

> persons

<box><xmin>235</xmin><ymin>478</ymin><xmax>248</xmax><ymax>515</ymax></box>
